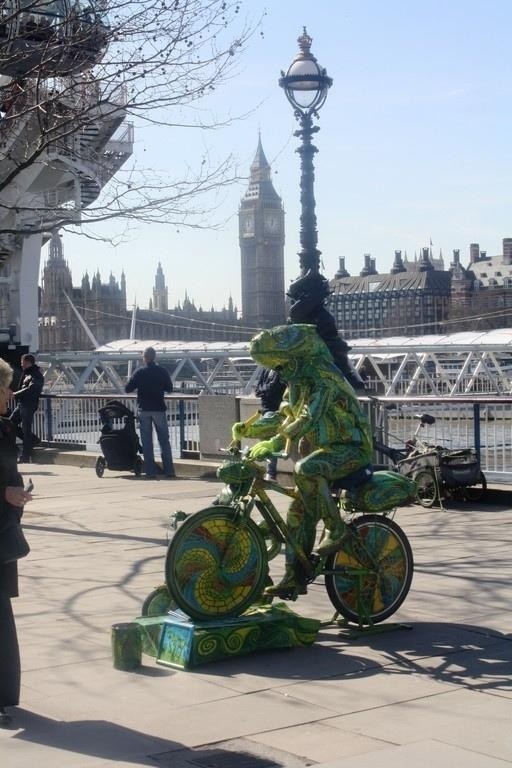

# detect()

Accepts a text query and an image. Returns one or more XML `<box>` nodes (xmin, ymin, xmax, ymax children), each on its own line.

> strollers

<box><xmin>95</xmin><ymin>400</ymin><xmax>144</xmax><ymax>477</ymax></box>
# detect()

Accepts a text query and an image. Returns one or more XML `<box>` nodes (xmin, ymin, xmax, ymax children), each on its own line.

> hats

<box><xmin>144</xmin><ymin>347</ymin><xmax>156</xmax><ymax>359</ymax></box>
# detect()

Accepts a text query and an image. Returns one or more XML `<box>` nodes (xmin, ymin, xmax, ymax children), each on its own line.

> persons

<box><xmin>123</xmin><ymin>347</ymin><xmax>179</xmax><ymax>480</ymax></box>
<box><xmin>0</xmin><ymin>357</ymin><xmax>35</xmax><ymax>731</ymax></box>
<box><xmin>228</xmin><ymin>318</ymin><xmax>372</xmax><ymax>602</ymax></box>
<box><xmin>9</xmin><ymin>352</ymin><xmax>46</xmax><ymax>466</ymax></box>
<box><xmin>253</xmin><ymin>364</ymin><xmax>288</xmax><ymax>483</ymax></box>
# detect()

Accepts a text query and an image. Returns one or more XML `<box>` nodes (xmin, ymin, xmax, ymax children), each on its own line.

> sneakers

<box><xmin>264</xmin><ymin>474</ymin><xmax>277</xmax><ymax>482</ymax></box>
<box><xmin>168</xmin><ymin>474</ymin><xmax>176</xmax><ymax>478</ymax></box>
<box><xmin>140</xmin><ymin>472</ymin><xmax>157</xmax><ymax>479</ymax></box>
<box><xmin>17</xmin><ymin>459</ymin><xmax>30</xmax><ymax>464</ymax></box>
<box><xmin>0</xmin><ymin>707</ymin><xmax>11</xmax><ymax>725</ymax></box>
<box><xmin>32</xmin><ymin>438</ymin><xmax>40</xmax><ymax>445</ymax></box>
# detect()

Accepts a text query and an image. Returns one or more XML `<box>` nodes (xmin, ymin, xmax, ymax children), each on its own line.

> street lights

<box><xmin>278</xmin><ymin>23</ymin><xmax>340</xmax><ymax>326</ymax></box>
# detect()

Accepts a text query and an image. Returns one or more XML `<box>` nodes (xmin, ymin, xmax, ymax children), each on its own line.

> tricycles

<box><xmin>387</xmin><ymin>438</ymin><xmax>488</xmax><ymax>511</ymax></box>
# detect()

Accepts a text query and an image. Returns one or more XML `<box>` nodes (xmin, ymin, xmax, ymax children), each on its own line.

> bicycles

<box><xmin>166</xmin><ymin>435</ymin><xmax>415</xmax><ymax>625</ymax></box>
<box><xmin>367</xmin><ymin>392</ymin><xmax>435</xmax><ymax>468</ymax></box>
